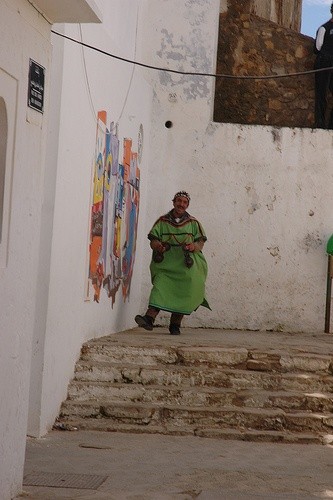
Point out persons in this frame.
[134,190,212,335]
[310,2,333,130]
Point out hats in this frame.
[173,190,190,202]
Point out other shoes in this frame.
[135,314,154,331]
[168,321,181,335]
